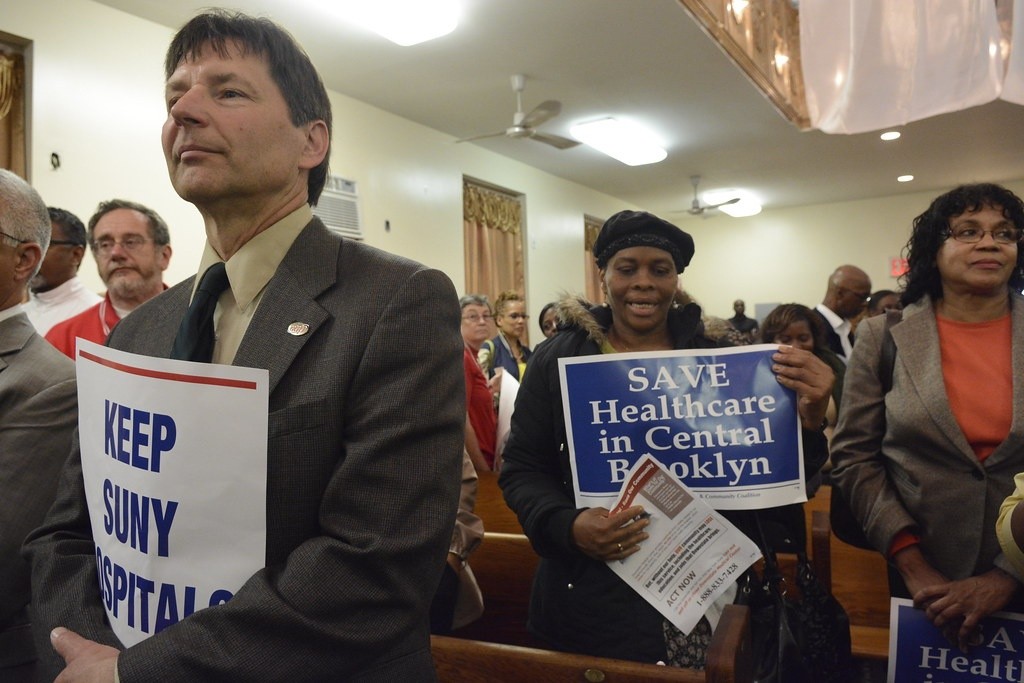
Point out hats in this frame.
[592,210,695,274]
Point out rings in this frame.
[617,543,623,553]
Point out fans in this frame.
[453,73,582,151]
[666,172,741,217]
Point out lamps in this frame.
[571,114,669,168]
[702,187,762,218]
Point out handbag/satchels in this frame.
[733,506,853,683]
[830,308,903,553]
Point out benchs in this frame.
[432,463,892,683]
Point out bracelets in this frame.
[818,417,829,433]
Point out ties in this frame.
[168,261,230,363]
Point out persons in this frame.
[0,168,77,683]
[45,199,172,358]
[539,303,563,338]
[869,290,902,318]
[50,6,464,683]
[476,291,533,416]
[764,303,846,409]
[431,443,485,641]
[813,264,872,360]
[502,209,834,668]
[23,206,105,337]
[730,301,757,336]
[825,182,1024,642]
[458,293,497,474]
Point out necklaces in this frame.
[619,342,631,352]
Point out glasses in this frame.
[507,313,529,320]
[462,313,495,322]
[93,236,155,250]
[948,225,1024,244]
